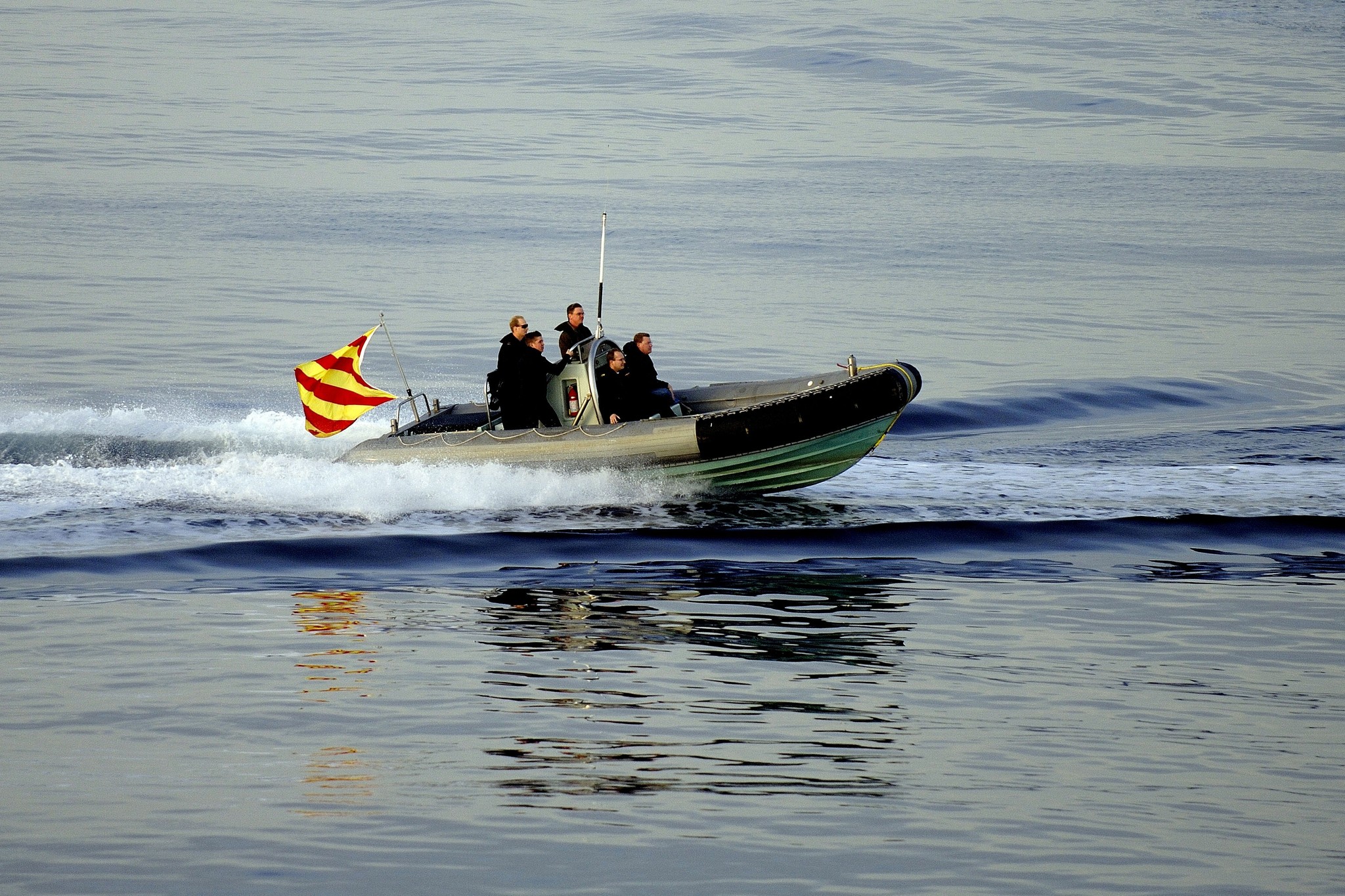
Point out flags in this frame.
[293,318,405,440]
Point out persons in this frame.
[559,303,593,364]
[597,332,694,425]
[496,315,573,431]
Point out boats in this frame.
[330,360,924,502]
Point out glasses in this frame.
[572,312,584,316]
[615,358,626,361]
[641,342,652,345]
[520,324,528,328]
